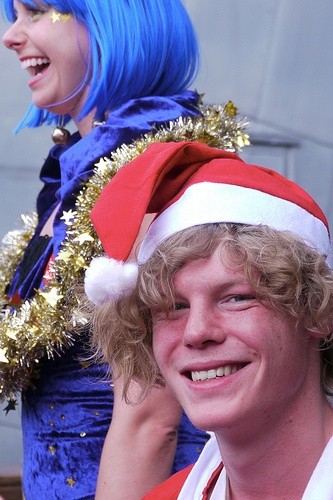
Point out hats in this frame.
[84,142,331,305]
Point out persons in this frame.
[83,141,332,500]
[0,0,252,500]
